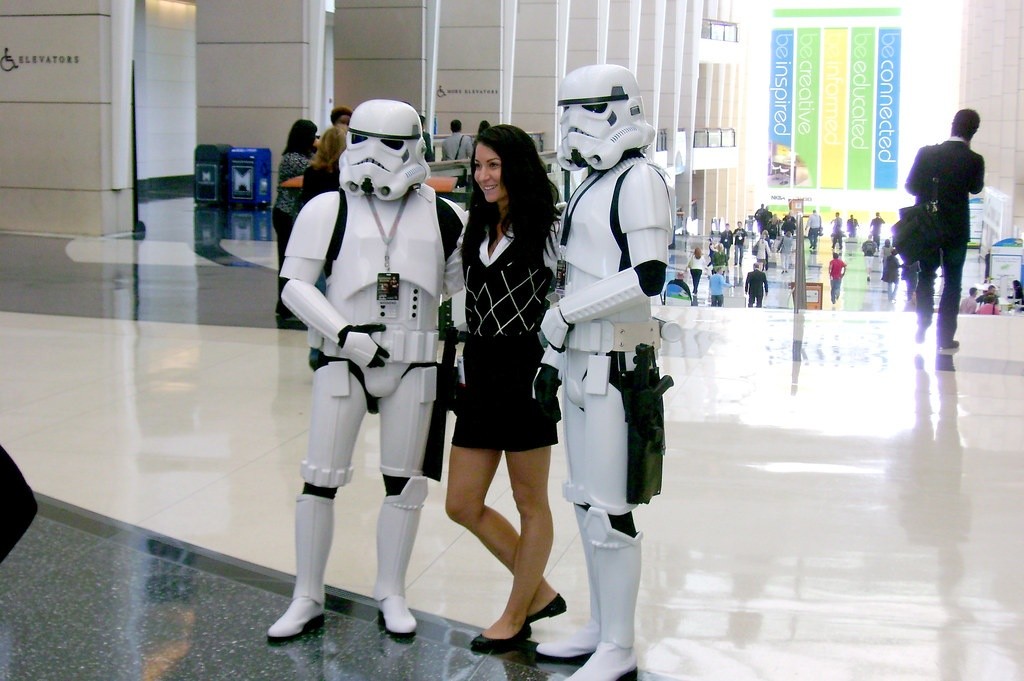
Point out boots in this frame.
[564,506,643,681]
[269,494,336,640]
[375,476,430,634]
[536,503,605,658]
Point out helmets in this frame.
[555,64,656,172]
[339,99,430,201]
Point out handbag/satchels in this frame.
[818,226,823,236]
[776,244,784,252]
[892,199,942,266]
[752,241,760,255]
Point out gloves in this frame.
[541,305,574,354]
[535,366,562,423]
[338,324,389,368]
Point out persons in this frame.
[478,120,491,134]
[829,252,847,304]
[719,223,733,255]
[560,267,565,285]
[862,234,878,284]
[847,215,858,237]
[870,212,885,251]
[530,63,674,681]
[831,212,842,249]
[882,239,1024,315]
[732,221,747,267]
[805,210,822,250]
[265,96,468,639]
[904,108,985,355]
[441,120,474,188]
[664,271,692,301]
[419,112,434,161]
[688,248,709,297]
[707,238,730,276]
[443,123,566,650]
[745,263,768,308]
[272,105,355,316]
[390,276,399,300]
[753,203,797,274]
[709,266,735,306]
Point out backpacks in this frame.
[865,242,874,256]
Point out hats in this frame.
[717,243,724,252]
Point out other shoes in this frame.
[275,301,295,319]
[916,316,933,343]
[471,622,532,648]
[938,339,960,349]
[525,592,567,623]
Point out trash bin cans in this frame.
[192,144,270,208]
